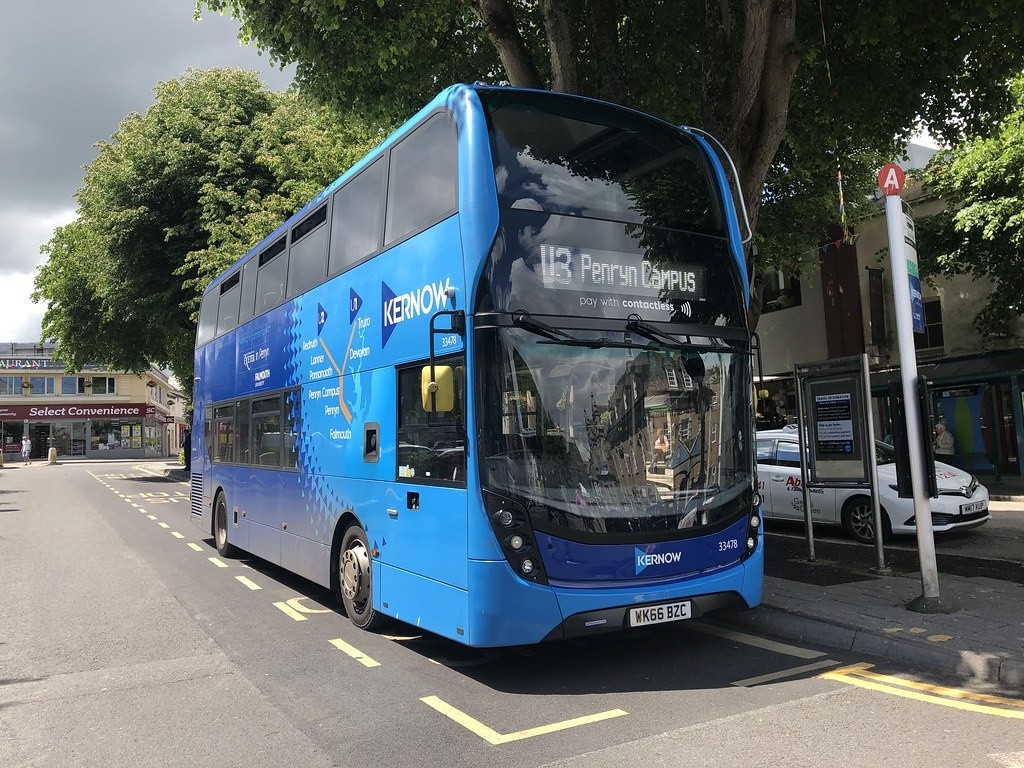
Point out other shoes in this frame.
[184,467,190,471]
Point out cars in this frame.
[755,425,993,543]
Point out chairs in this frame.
[397,427,574,493]
[261,432,299,469]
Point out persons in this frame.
[181,429,191,471]
[933,424,955,465]
[649,429,669,473]
[21,436,32,465]
[787,416,794,425]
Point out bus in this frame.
[189,81,766,648]
